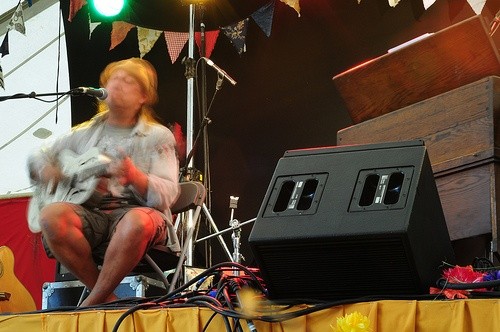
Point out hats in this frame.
[100,59,156,96]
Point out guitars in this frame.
[26,149,131,234]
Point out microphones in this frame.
[78,87,108,101]
[204,57,238,86]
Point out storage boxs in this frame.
[332,15,500,241]
[41,275,171,312]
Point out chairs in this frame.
[76,180,233,307]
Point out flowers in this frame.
[331,313,377,332]
[440,265,500,299]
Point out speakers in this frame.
[246,139,456,307]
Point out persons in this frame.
[26,58,182,308]
